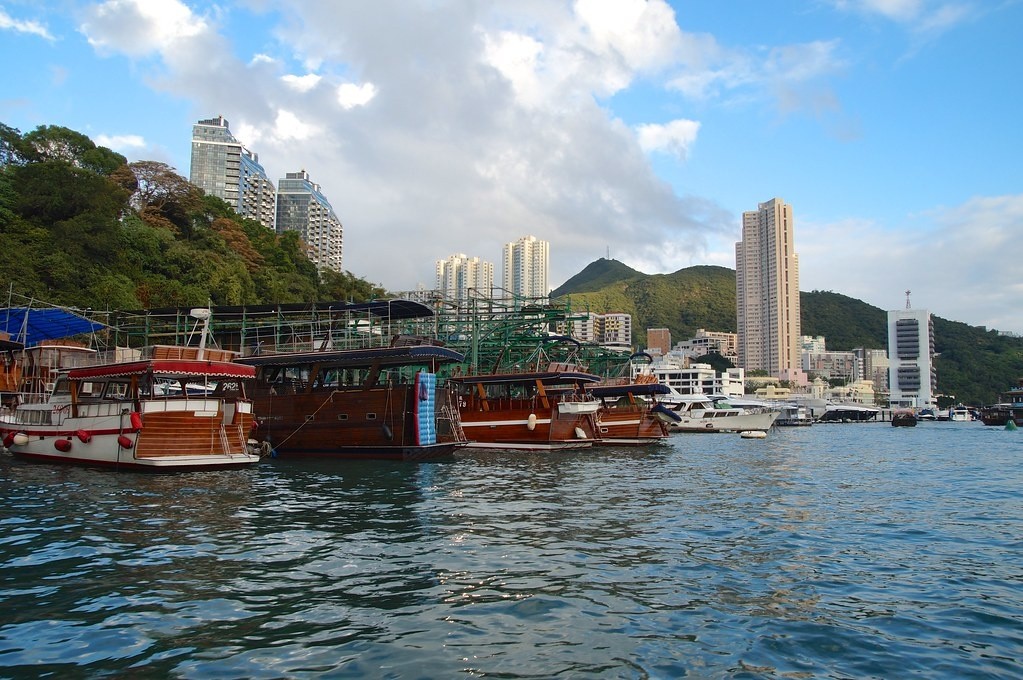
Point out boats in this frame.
[892,409,917,428]
[0,298,262,473]
[116,297,880,461]
[918,387,1023,426]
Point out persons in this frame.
[391,368,408,384]
[441,364,473,389]
[894,414,910,418]
